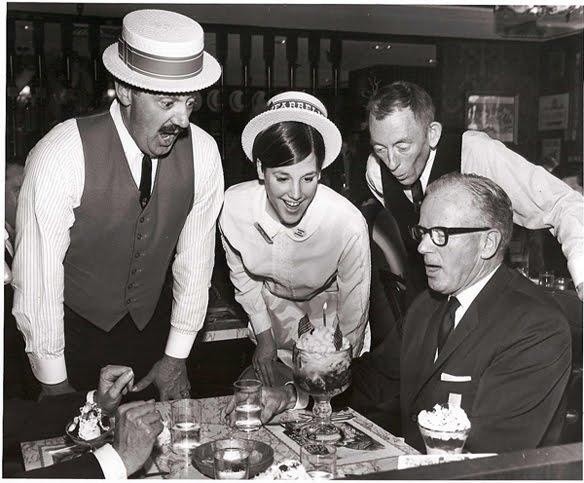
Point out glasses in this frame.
[407,224,492,247]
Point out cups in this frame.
[170,397,200,457]
[539,272,555,289]
[299,444,339,479]
[416,423,471,456]
[233,379,263,429]
[214,448,247,479]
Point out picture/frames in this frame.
[536,90,571,133]
[539,136,563,161]
[464,90,519,146]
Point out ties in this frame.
[410,178,424,207]
[437,295,461,355]
[139,154,152,210]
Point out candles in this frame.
[322,302,327,327]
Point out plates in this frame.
[191,438,275,478]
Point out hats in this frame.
[102,9,222,94]
[241,90,343,171]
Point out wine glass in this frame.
[293,343,352,445]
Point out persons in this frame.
[329,172,574,458]
[2,13,226,481]
[217,91,372,411]
[364,79,583,309]
